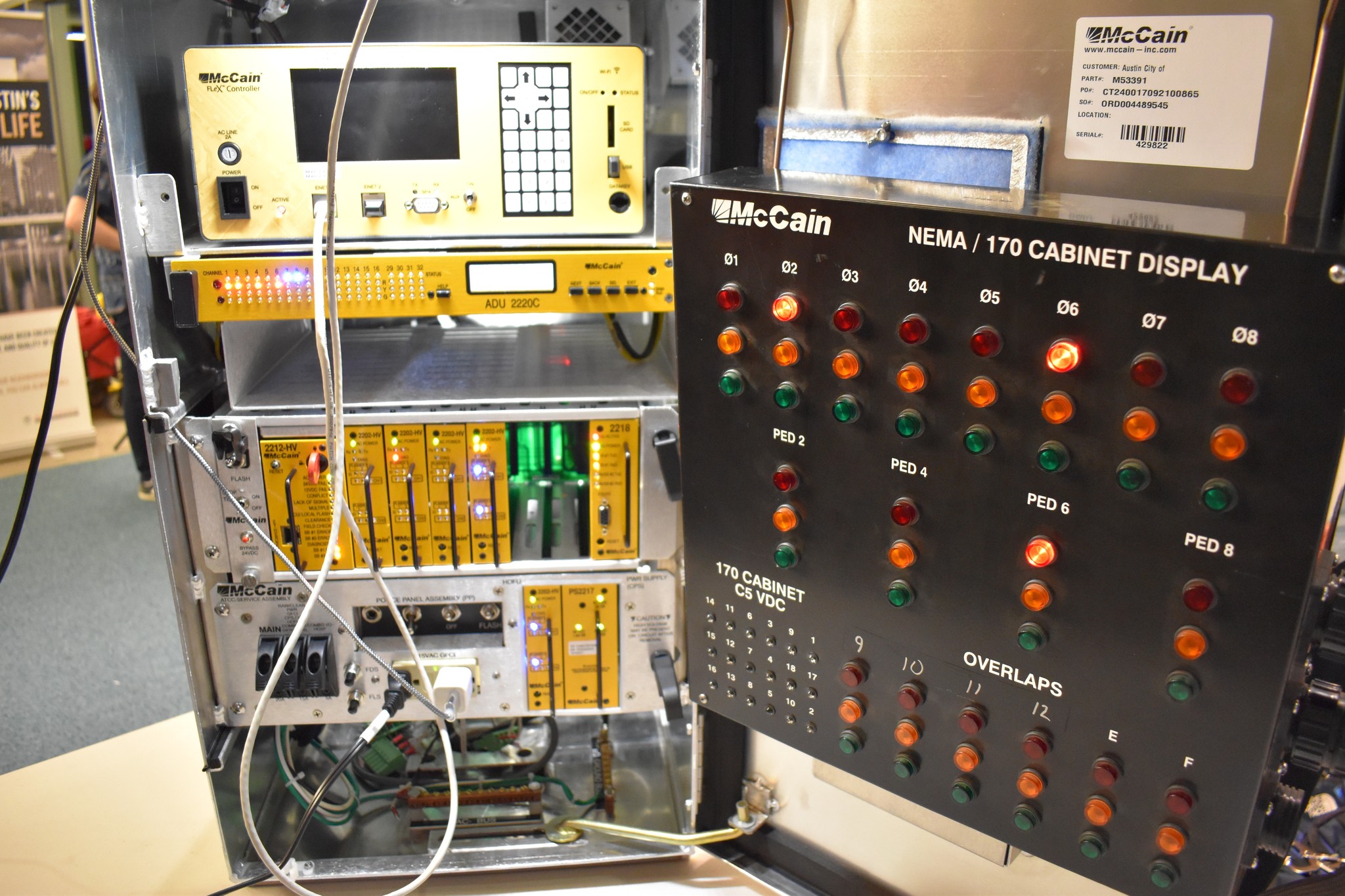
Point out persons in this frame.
[63,75,159,502]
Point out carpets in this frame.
[0,454,194,777]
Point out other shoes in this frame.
[138,483,156,502]
